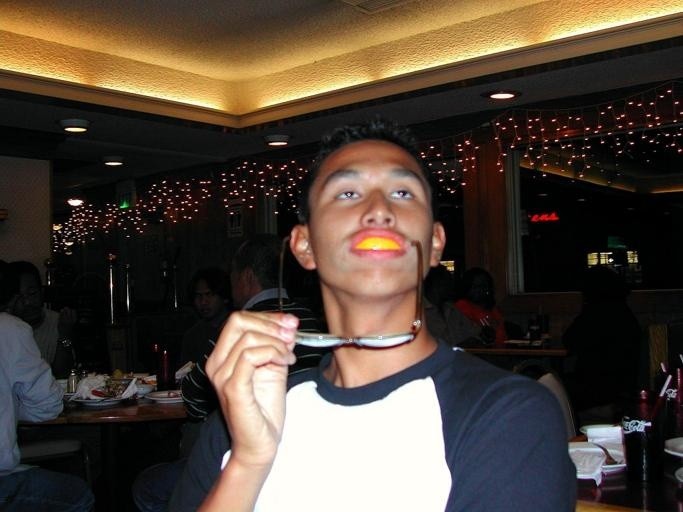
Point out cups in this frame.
[623,389,670,483]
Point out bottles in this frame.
[67,369,89,393]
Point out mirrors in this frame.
[504,123,683,293]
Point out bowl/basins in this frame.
[579,424,621,441]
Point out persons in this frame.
[423,263,512,373]
[563,268,638,412]
[1,237,326,512]
[167,129,578,512]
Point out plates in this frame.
[565,442,626,478]
[133,383,153,399]
[72,397,121,407]
[143,389,184,405]
[663,434,683,460]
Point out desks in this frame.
[13,329,683,512]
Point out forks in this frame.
[589,441,624,466]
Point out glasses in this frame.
[278,235,424,349]
